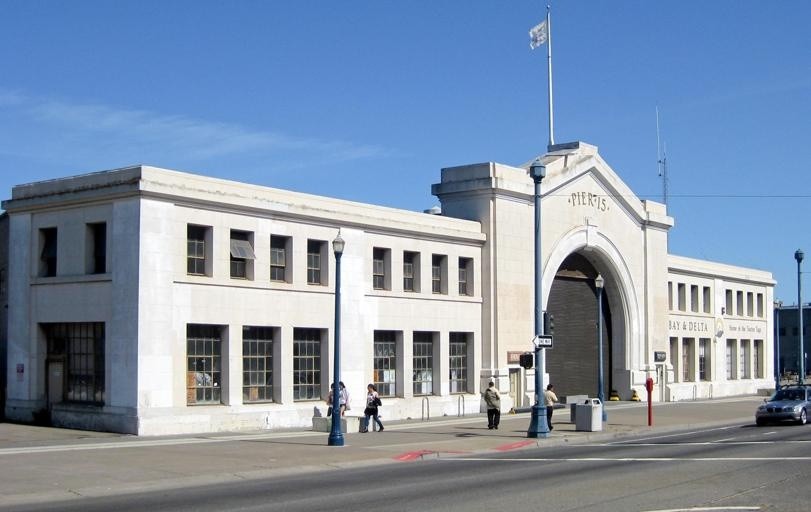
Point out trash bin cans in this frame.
[575,398,602,431]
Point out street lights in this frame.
[330,227,345,447]
[528,156,549,436]
[794,248,805,384]
[594,273,607,423]
[772,299,784,391]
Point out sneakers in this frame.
[362,425,384,432]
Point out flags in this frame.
[528,19,548,50]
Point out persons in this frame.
[483,381,501,429]
[339,381,352,417]
[362,383,384,433]
[544,384,559,431]
[326,383,333,416]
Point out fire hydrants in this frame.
[646,377,653,427]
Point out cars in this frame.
[757,384,811,425]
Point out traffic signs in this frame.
[533,336,552,350]
